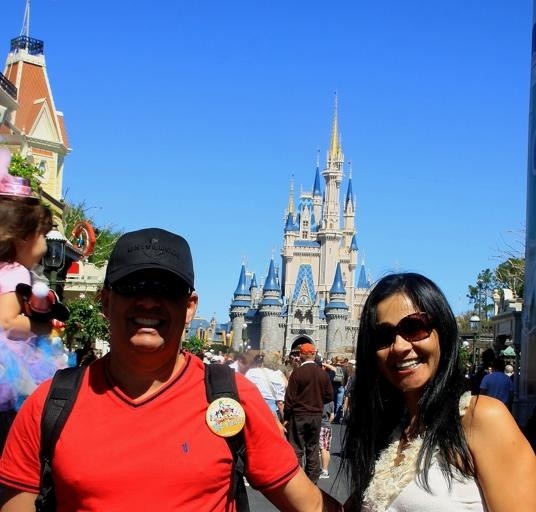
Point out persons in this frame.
[284,343,356,484]
[339,273,536,512]
[0,194,68,412]
[0,228,344,512]
[466,360,520,405]
[195,348,298,419]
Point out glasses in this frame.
[110,270,191,301]
[366,313,432,350]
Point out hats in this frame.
[300,342,313,356]
[106,229,194,293]
